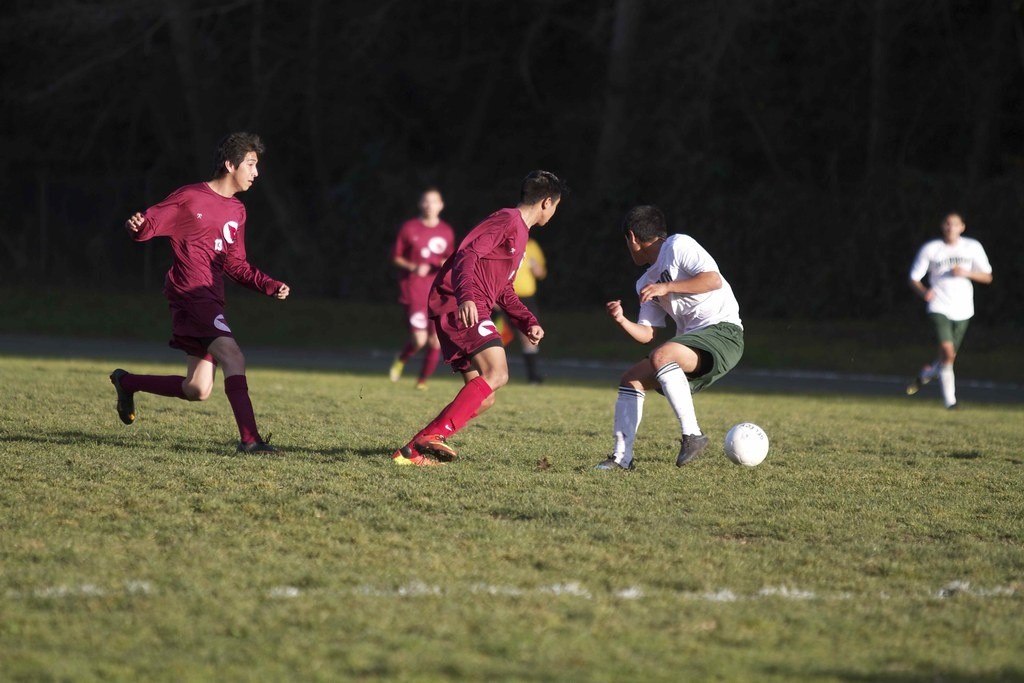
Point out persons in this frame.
[387,186,454,390]
[110,132,285,457]
[595,205,744,471]
[489,234,547,385]
[905,210,994,412]
[392,169,562,467]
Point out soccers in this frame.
[724,422,770,467]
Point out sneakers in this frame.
[236,432,279,455]
[414,434,457,462]
[906,366,933,396]
[947,403,963,410]
[390,449,447,467]
[676,429,710,466]
[389,352,405,382]
[110,368,136,425]
[415,383,427,390]
[594,454,636,471]
